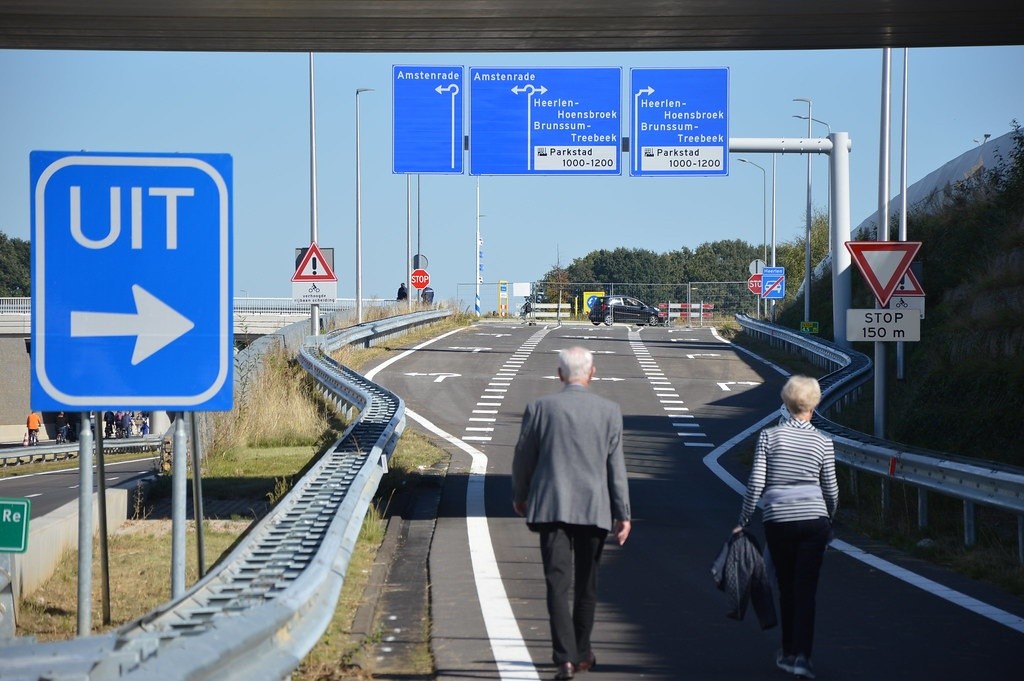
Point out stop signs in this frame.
[747,273,763,295]
[410,269,431,290]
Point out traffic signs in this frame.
[389,63,467,174]
[466,66,625,177]
[628,64,730,178]
[28,149,236,414]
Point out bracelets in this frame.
[738,521,752,528]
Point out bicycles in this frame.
[102,418,134,439]
[54,425,71,445]
[516,307,536,326]
[26,424,41,447]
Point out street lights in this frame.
[792,97,813,338]
[793,113,832,255]
[355,87,377,324]
[736,157,768,318]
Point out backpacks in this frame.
[525,304,531,312]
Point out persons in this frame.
[396,282,407,300]
[26,410,151,445]
[492,311,497,316]
[730,373,840,675]
[511,347,632,681]
[522,298,531,323]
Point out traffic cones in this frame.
[22,432,29,447]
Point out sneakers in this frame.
[794,658,816,680]
[776,656,794,672]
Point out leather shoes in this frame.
[575,651,595,672]
[554,663,574,680]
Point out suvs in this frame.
[588,294,663,327]
